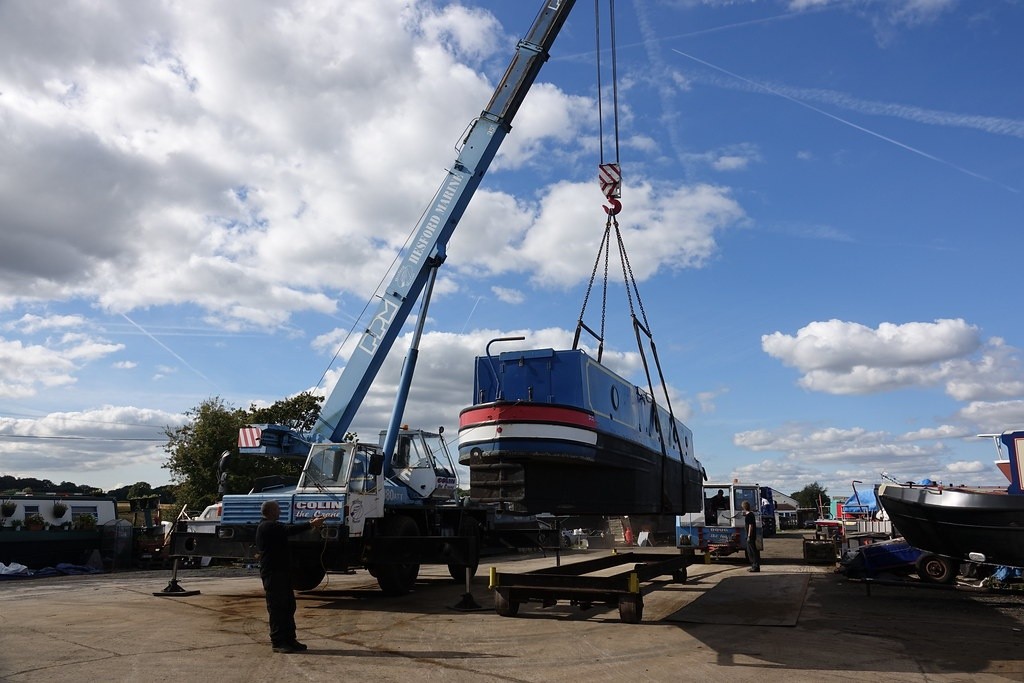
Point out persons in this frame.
[741,500,760,573]
[256,501,323,654]
[708,489,728,512]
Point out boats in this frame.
[873,483,1024,570]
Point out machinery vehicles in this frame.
[154,0,625,600]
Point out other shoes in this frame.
[747,568,760,572]
[290,639,307,652]
[271,643,295,653]
